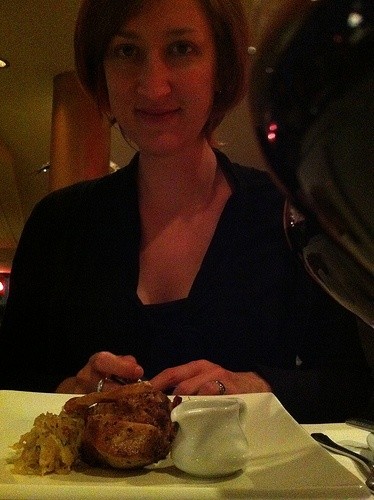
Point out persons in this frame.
[2,1,370,424]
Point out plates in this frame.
[1,389,373,499]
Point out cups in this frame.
[170,397,254,481]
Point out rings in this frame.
[213,379,226,395]
[96,377,109,392]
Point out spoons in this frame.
[310,431,374,495]
